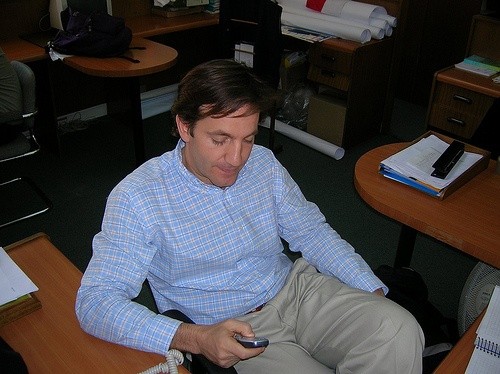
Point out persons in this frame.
[74,60,424,374]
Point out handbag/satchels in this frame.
[373,265,427,326]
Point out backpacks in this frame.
[51,10,132,57]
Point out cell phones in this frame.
[232,334,270,349]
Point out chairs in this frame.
[0,61,54,228]
[220,0,287,153]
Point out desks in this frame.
[0,13,219,165]
[352,142,500,374]
[0,232,191,374]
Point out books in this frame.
[0,247,41,324]
[453,54,500,77]
[465,285,500,374]
[375,136,485,200]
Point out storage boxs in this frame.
[306,96,356,146]
[140,76,182,119]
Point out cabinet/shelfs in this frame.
[308,37,393,130]
[424,65,500,142]
